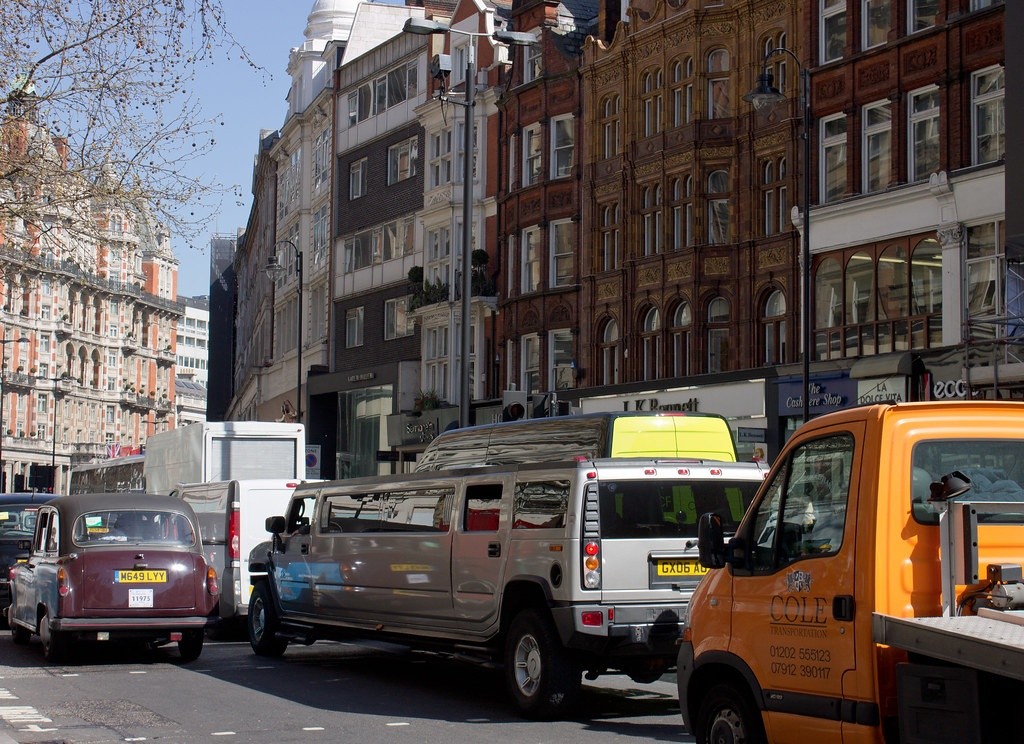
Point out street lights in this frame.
[403,17,538,429]
[50,377,76,493]
[260,240,304,423]
[0,331,30,493]
[742,48,813,497]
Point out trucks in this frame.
[142,422,305,495]
[677,401,1024,744]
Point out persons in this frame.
[291,525,310,536]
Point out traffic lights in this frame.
[48,484,53,494]
[502,383,528,422]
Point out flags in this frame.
[107,444,120,458]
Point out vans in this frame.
[414,411,739,475]
[247,458,771,711]
[168,479,332,640]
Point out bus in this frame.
[68,455,148,524]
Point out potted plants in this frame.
[413,384,441,411]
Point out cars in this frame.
[0,493,220,663]
